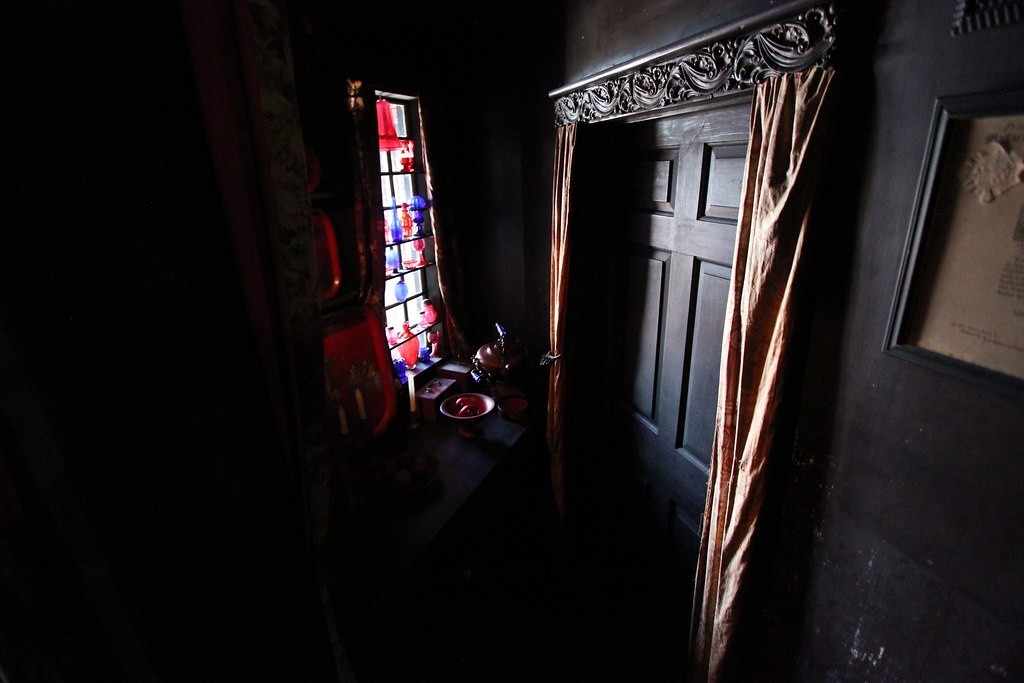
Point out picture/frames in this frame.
[882,82,1023,402]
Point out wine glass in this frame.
[426,331,441,358]
[415,239,427,265]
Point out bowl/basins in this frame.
[401,260,418,269]
[498,395,528,421]
[385,267,394,278]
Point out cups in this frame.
[402,139,415,172]
[394,359,408,379]
[420,347,430,362]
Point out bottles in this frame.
[396,323,419,370]
[389,195,412,242]
[386,327,398,345]
[418,299,437,328]
[387,246,400,269]
[396,280,408,303]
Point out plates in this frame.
[443,394,495,437]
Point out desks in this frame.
[320,417,530,605]
